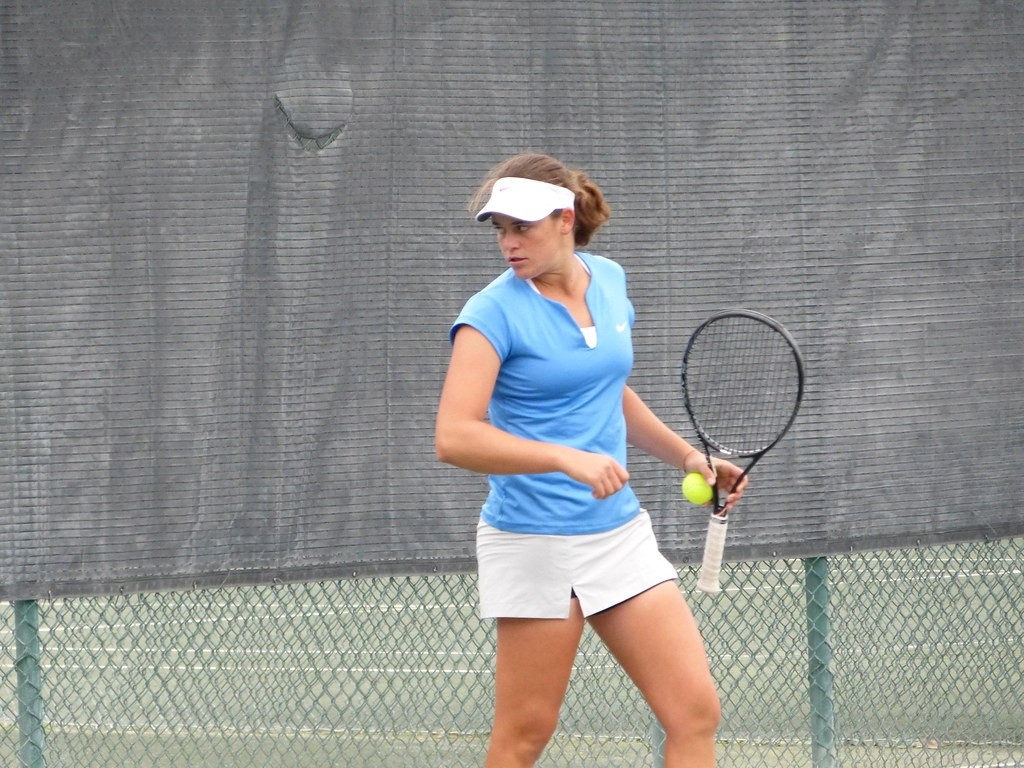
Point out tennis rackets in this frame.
[679,310,806,594]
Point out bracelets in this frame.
[679,449,701,473]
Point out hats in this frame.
[474,177,575,223]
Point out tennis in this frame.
[683,473,712,506]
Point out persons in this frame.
[435,154,751,768]
